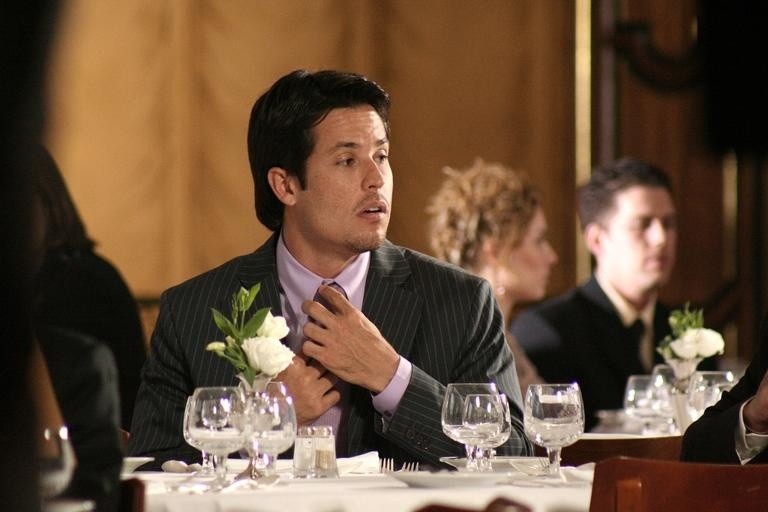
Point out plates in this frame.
[390,465,527,488]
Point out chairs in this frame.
[556,436,692,472]
[586,457,767,510]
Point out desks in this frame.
[119,458,594,511]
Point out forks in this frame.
[380,456,420,475]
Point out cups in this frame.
[623,363,734,435]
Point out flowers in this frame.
[205,282,296,393]
[656,300,725,381]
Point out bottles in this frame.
[293,425,339,478]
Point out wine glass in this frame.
[462,392,513,473]
[182,380,298,482]
[524,381,586,478]
[441,380,498,472]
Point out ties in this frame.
[629,320,644,351]
[309,281,348,314]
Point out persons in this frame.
[423,158,556,424]
[0,139,147,437]
[511,157,721,434]
[128,66,533,471]
[679,336,767,467]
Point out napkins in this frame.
[226,451,380,478]
